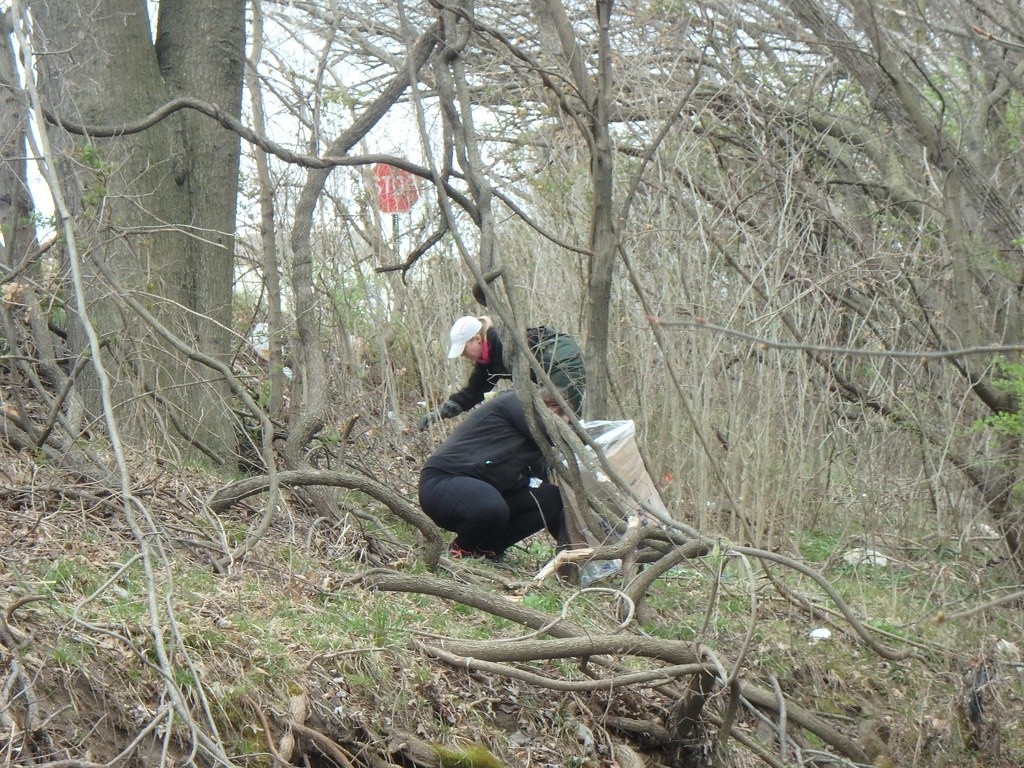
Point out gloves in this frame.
[420,400,462,432]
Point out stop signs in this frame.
[372,160,424,215]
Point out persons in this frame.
[418,386,572,558]
[417,316,587,433]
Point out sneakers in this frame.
[448,540,514,575]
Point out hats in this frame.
[447,316,482,359]
